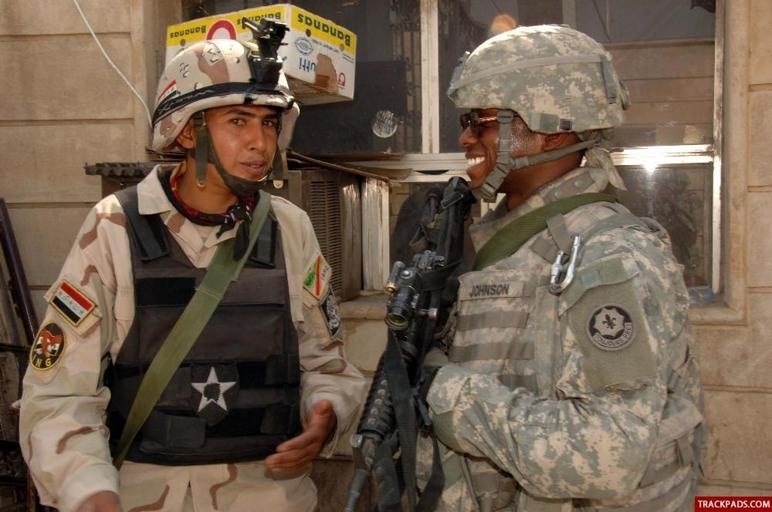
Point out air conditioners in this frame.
[101,169,362,304]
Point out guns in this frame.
[344,176,477,512]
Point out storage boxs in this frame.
[165,3,357,106]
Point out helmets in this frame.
[149,38,300,154]
[447,23,632,136]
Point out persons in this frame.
[17,35,368,511]
[412,21,707,511]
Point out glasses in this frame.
[459,112,521,138]
